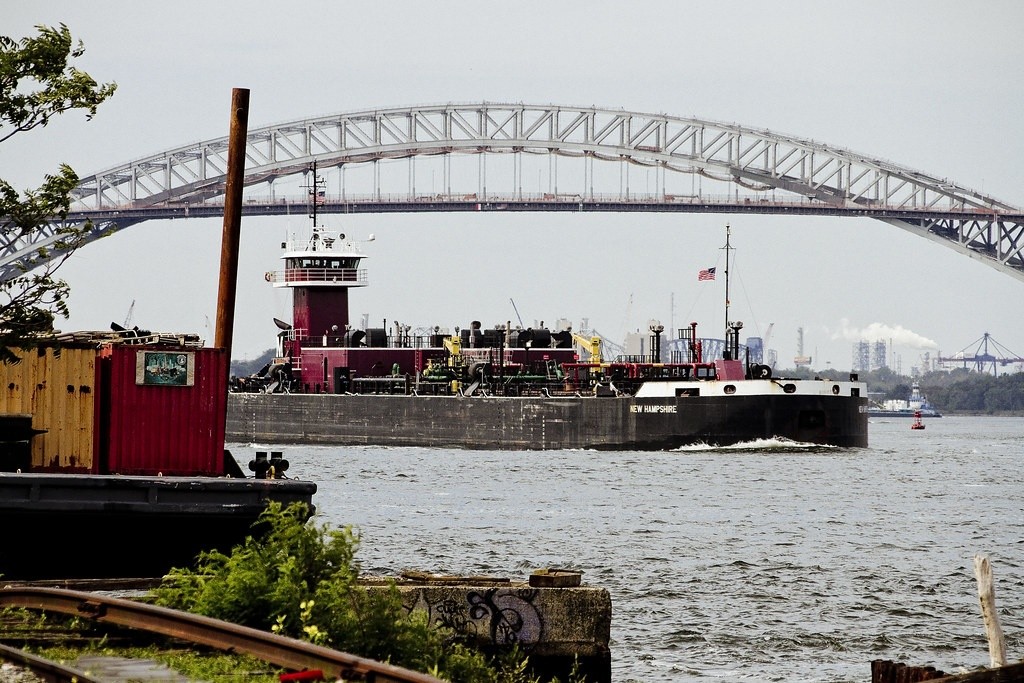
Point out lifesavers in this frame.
[265,272,270,281]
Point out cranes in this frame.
[763,323,775,352]
[123,300,135,331]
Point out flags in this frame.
[698,267,716,281]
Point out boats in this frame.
[0,86,316,580]
[867,376,942,417]
[225,161,869,452]
[912,408,926,429]
